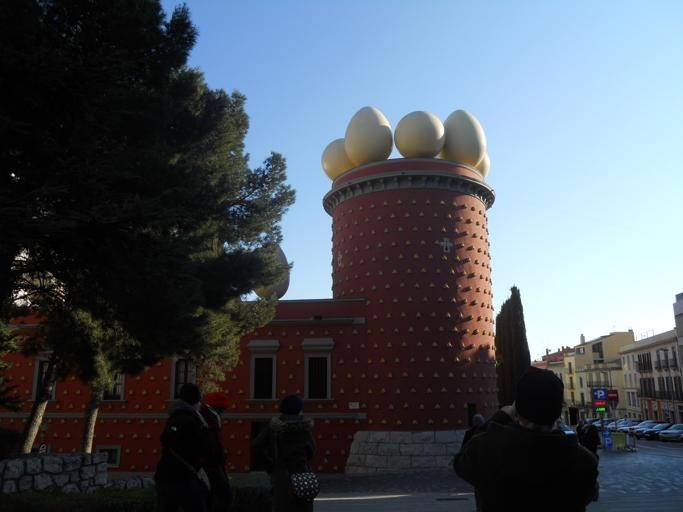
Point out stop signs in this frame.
[607,389,618,401]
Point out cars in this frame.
[561,423,578,443]
[582,416,682,444]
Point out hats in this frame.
[200,391,232,411]
[512,364,565,427]
[276,394,304,414]
[173,382,201,405]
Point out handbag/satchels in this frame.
[284,469,322,504]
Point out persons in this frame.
[451,368,599,512]
[270,394,319,512]
[581,419,601,461]
[151,382,213,511]
[576,419,583,445]
[460,412,485,449]
[196,392,237,512]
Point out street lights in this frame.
[632,359,646,419]
[599,365,620,451]
[657,347,677,423]
